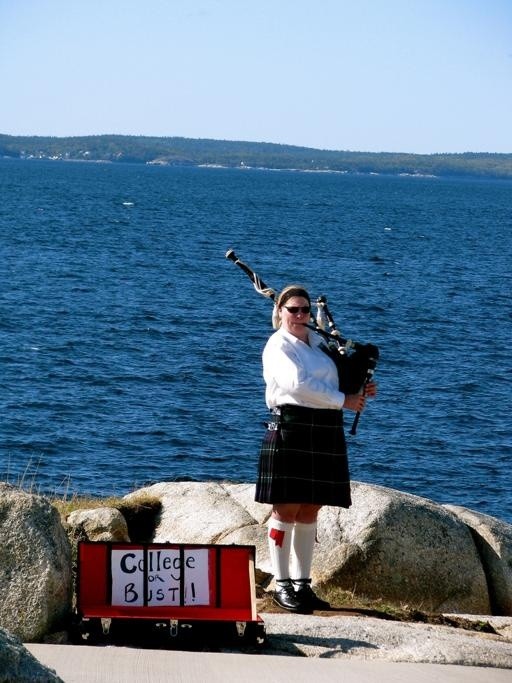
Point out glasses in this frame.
[282,304,311,314]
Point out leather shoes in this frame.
[272,583,330,614]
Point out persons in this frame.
[255,287,376,611]
